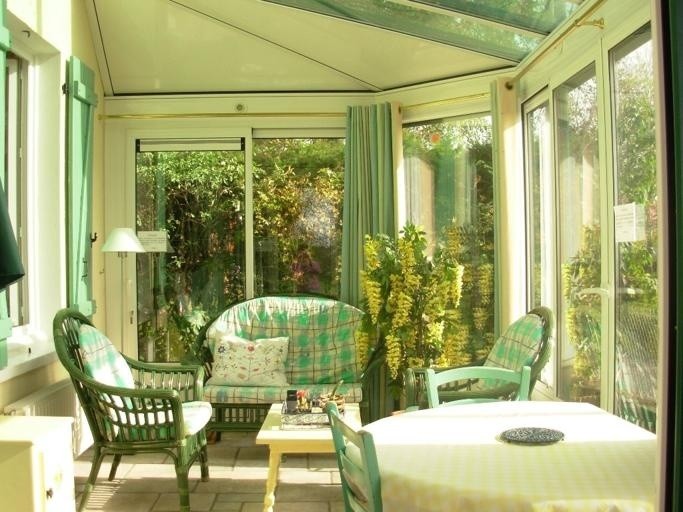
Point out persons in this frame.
[292,251,322,293]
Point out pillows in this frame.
[212,328,291,386]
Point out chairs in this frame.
[403,306,552,411]
[322,400,382,511]
[52,306,213,510]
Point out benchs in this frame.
[196,295,381,443]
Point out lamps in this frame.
[99,227,147,356]
